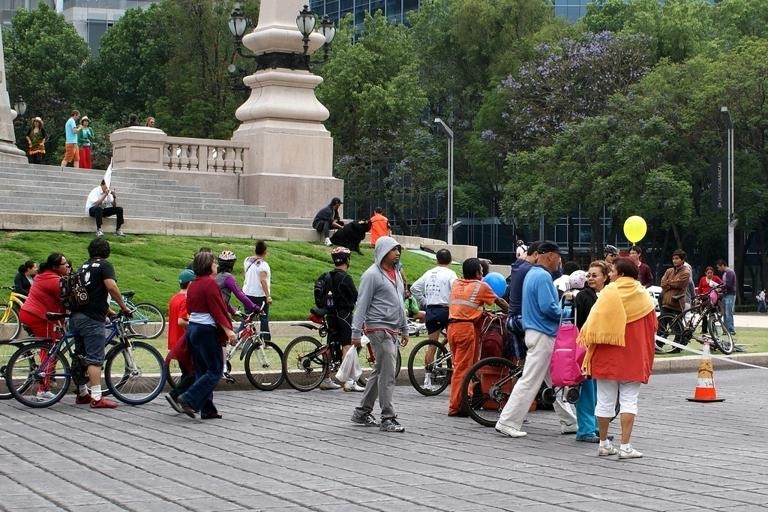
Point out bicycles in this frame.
[0,284,38,345]
[171,309,284,391]
[652,293,734,354]
[279,310,400,392]
[463,330,525,427]
[106,289,164,339]
[407,314,504,395]
[6,310,166,407]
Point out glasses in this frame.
[586,274,599,278]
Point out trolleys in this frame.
[539,292,586,404]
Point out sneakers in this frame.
[75,394,91,404]
[576,435,599,443]
[344,382,366,392]
[165,392,183,412]
[598,445,618,456]
[494,420,527,437]
[324,239,332,246]
[90,396,118,408]
[561,423,577,433]
[35,391,56,402]
[177,399,196,418]
[116,230,126,237]
[380,417,405,432]
[351,410,380,427]
[618,448,642,458]
[96,229,104,237]
[320,382,341,389]
[201,413,222,419]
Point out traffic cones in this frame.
[686,338,725,404]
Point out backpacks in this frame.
[481,316,504,367]
[60,258,89,309]
[314,271,335,309]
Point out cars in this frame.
[742,283,753,300]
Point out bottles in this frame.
[327,291,334,307]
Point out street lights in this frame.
[716,105,739,315]
[431,118,456,244]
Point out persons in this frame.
[329,219,372,254]
[397,234,738,458]
[78,114,96,168]
[124,113,141,128]
[86,176,125,239]
[24,116,50,162]
[13,236,134,410]
[365,206,392,248]
[348,235,410,434]
[163,240,276,419]
[316,245,367,395]
[146,115,155,128]
[751,280,768,313]
[312,196,348,245]
[60,106,81,167]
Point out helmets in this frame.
[218,250,237,260]
[331,247,352,259]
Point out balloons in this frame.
[621,214,648,251]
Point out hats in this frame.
[79,116,91,123]
[605,245,620,255]
[538,241,569,255]
[32,117,44,127]
[178,269,197,283]
[569,270,587,289]
